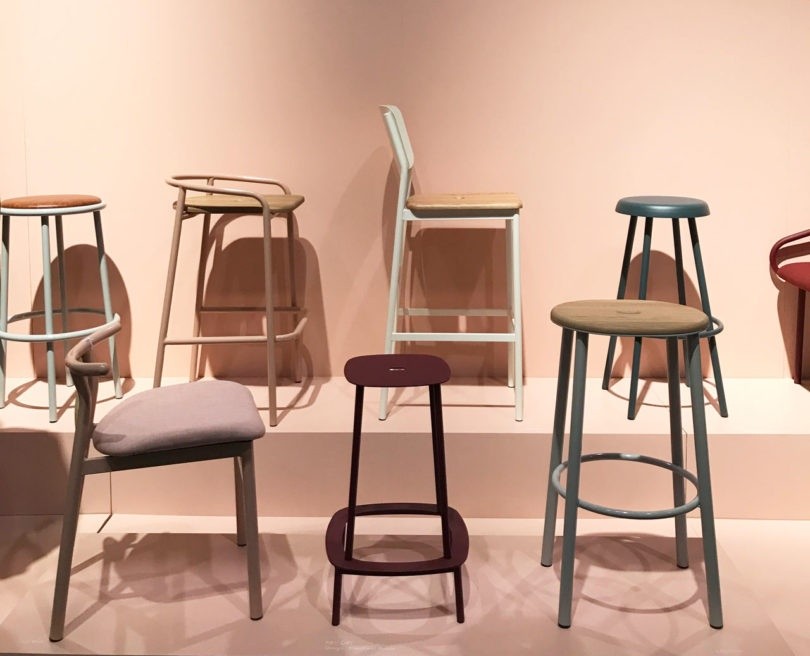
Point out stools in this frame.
[48,320,267,641]
[0,194,122,423]
[151,172,308,426]
[600,195,730,420]
[323,352,470,625]
[770,229,810,384]
[540,299,725,630]
[378,103,523,422]
